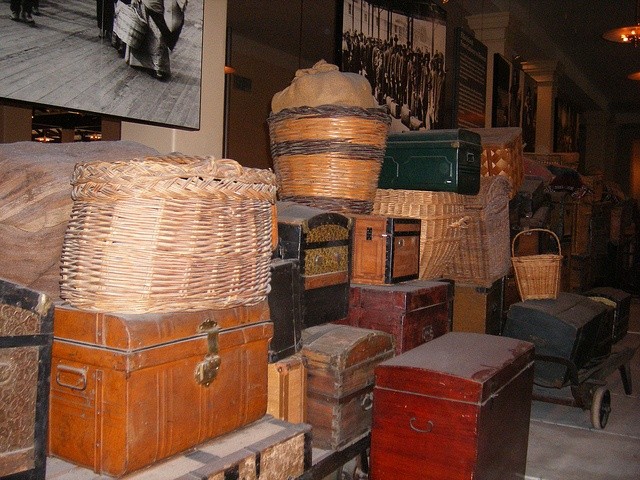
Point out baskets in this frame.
[512,228,564,302]
[583,174,601,200]
[60,158,278,309]
[112,1,148,51]
[372,190,472,282]
[267,109,391,214]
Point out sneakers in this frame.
[22,13,33,27]
[8,9,19,22]
[32,7,40,15]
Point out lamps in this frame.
[602,19,640,50]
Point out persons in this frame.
[21,0,40,19]
[557,116,576,152]
[124,0,187,82]
[341,30,446,131]
[9,0,35,22]
[523,87,535,151]
[97,0,131,58]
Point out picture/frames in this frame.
[0,0,204,132]
[336,0,447,129]
[491,54,539,154]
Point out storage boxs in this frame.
[0,280,53,479]
[368,332,536,480]
[275,200,353,328]
[268,259,304,363]
[343,213,421,285]
[300,324,396,453]
[48,301,276,477]
[204,356,312,479]
[325,280,451,357]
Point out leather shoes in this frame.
[152,69,168,81]
